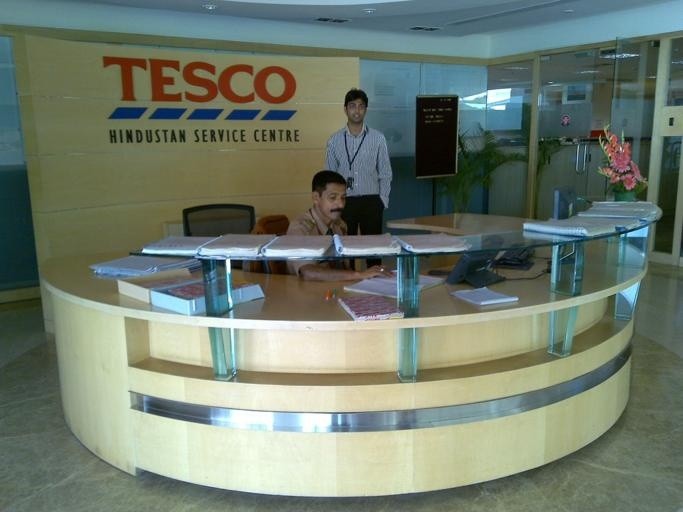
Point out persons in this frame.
[280,168,396,283]
[324,87,394,270]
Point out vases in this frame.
[615,188,636,203]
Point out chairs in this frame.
[181,202,290,276]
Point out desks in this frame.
[37,194,661,499]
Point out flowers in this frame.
[596,128,647,192]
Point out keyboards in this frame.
[497,241,536,265]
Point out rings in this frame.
[378,268,385,272]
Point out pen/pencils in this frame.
[325,287,337,301]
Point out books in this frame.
[339,294,406,323]
[85,254,204,281]
[341,268,447,298]
[447,284,520,308]
[520,199,657,238]
